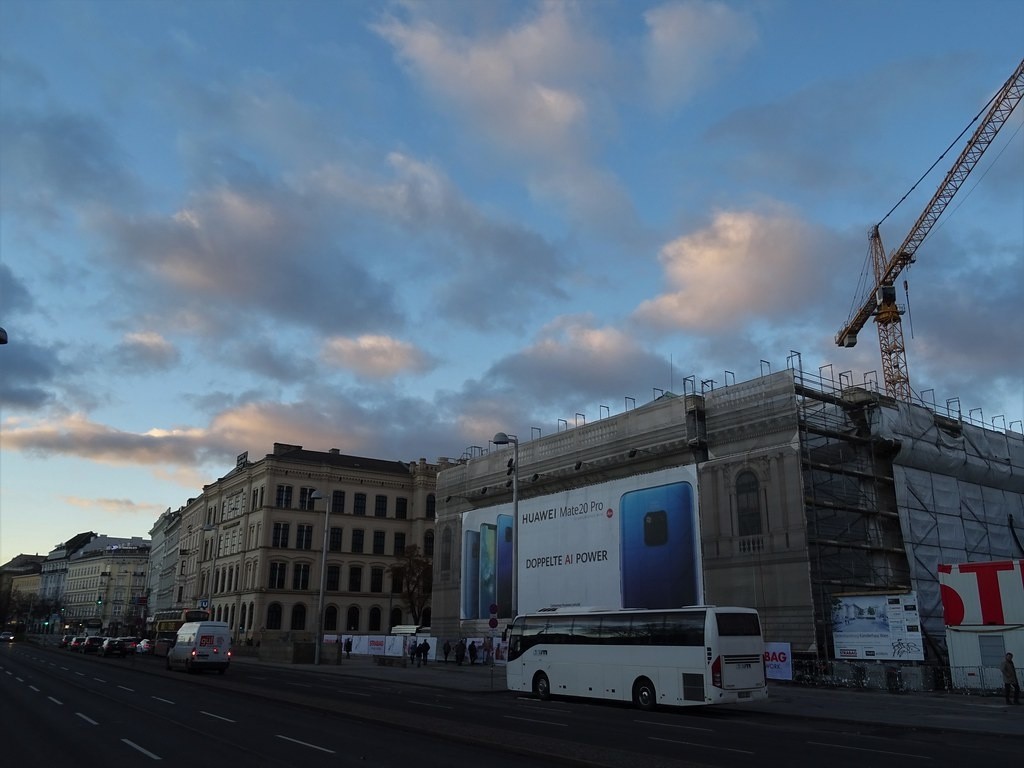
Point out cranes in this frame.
[834,58,1024,404]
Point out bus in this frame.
[82,618,109,637]
[502,606,769,710]
[151,609,211,656]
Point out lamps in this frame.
[445,496,466,502]
[629,448,659,458]
[532,474,549,481]
[575,461,601,470]
[506,480,524,487]
[481,487,502,494]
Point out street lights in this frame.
[311,490,330,641]
[203,525,220,610]
[494,432,520,621]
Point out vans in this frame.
[167,621,230,674]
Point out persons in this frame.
[496,643,507,660]
[443,640,451,664]
[346,638,352,659]
[85,633,88,637]
[106,633,109,637]
[410,639,430,668]
[455,640,466,666]
[1001,653,1022,705]
[468,641,477,664]
[95,632,99,636]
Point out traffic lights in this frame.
[97,600,102,605]
[60,608,65,613]
[44,620,49,626]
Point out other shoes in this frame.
[1006,700,1012,705]
[1014,699,1021,705]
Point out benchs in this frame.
[375,656,411,668]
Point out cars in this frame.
[68,637,86,652]
[0,632,14,641]
[136,638,154,654]
[61,635,76,648]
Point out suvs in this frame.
[78,636,104,655]
[96,639,127,659]
[117,636,137,655]
